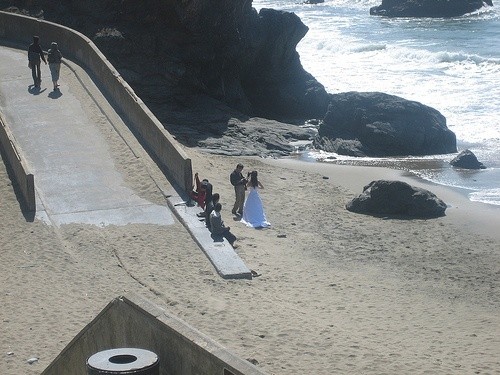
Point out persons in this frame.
[230,164,247,216]
[47,41,61,91]
[28,36,47,90]
[241,171,271,227]
[190,172,236,242]
